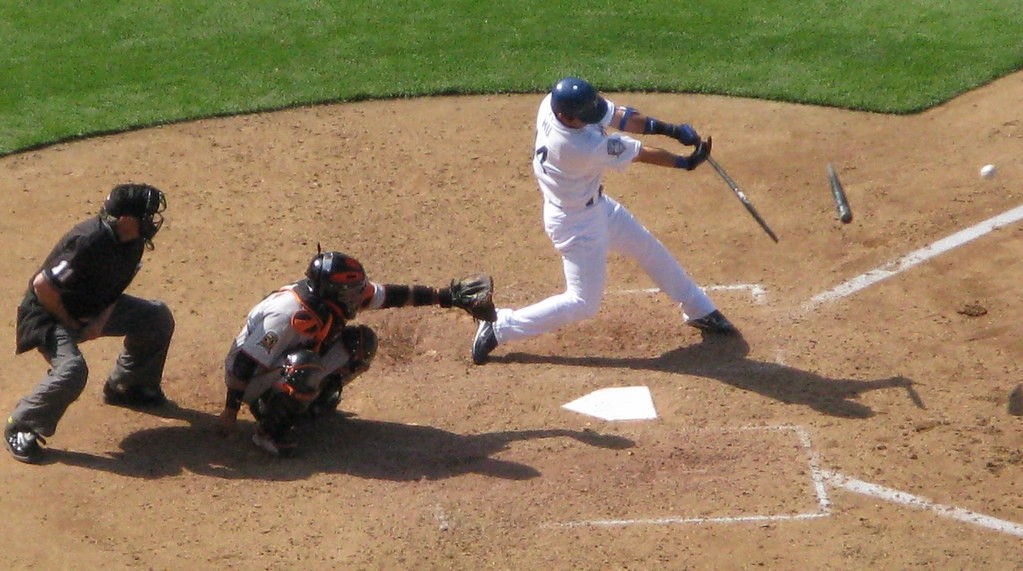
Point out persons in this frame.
[220,251,498,460]
[472,78,735,365]
[3,183,175,464]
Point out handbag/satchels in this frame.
[15,305,57,355]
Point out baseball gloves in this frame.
[439,273,498,323]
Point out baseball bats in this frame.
[695,143,780,244]
[826,161,853,224]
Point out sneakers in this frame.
[3,419,46,462]
[472,308,499,365]
[687,309,737,333]
[252,436,294,459]
[310,402,336,418]
[103,377,178,412]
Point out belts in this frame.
[549,185,601,211]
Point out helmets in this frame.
[304,251,367,320]
[550,77,608,124]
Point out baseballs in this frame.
[981,164,997,180]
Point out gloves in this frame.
[667,123,700,146]
[684,136,713,171]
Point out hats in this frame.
[104,184,159,217]
